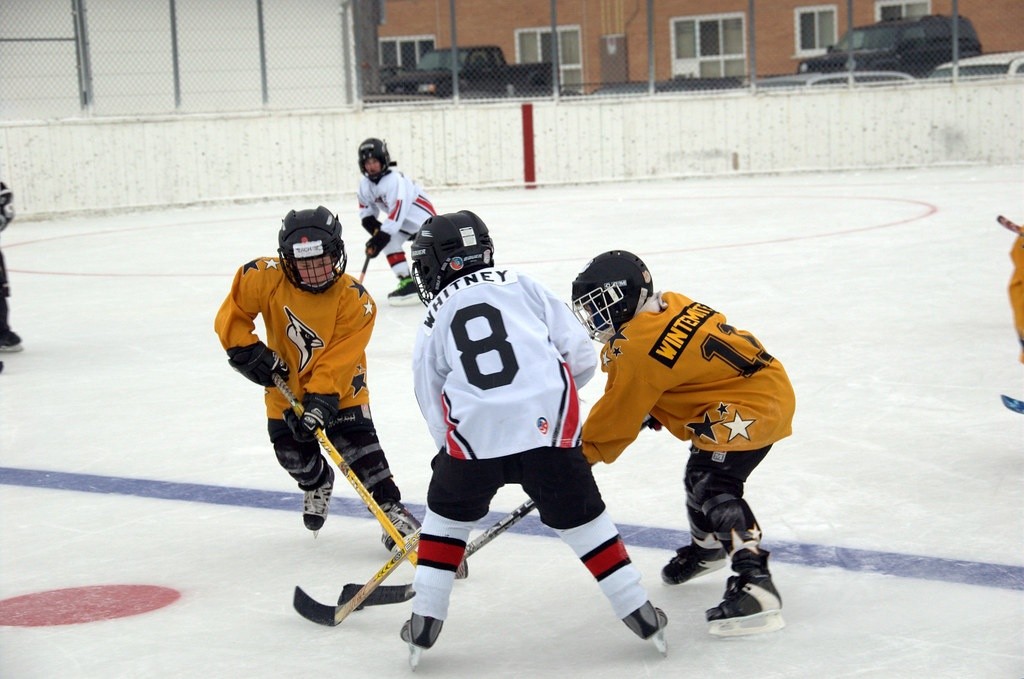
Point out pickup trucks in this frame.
[380,43,553,97]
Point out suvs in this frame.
[797,11,983,79]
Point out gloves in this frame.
[362,214,382,237]
[281,393,340,443]
[227,340,290,387]
[367,229,391,258]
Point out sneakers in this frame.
[402,610,443,671]
[622,603,669,653]
[704,550,785,637]
[388,272,422,305]
[366,500,421,556]
[298,458,334,541]
[0,328,21,353]
[660,540,728,594]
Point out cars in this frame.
[589,50,1024,95]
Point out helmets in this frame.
[279,207,345,293]
[571,250,653,346]
[357,140,389,178]
[410,210,495,305]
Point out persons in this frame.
[998,209,1024,369]
[0,181,23,375]
[357,138,438,306]
[214,206,423,552]
[400,209,668,669]
[564,247,795,638]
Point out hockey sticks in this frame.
[293,527,421,627]
[274,373,468,579]
[357,259,370,285]
[337,413,654,608]
